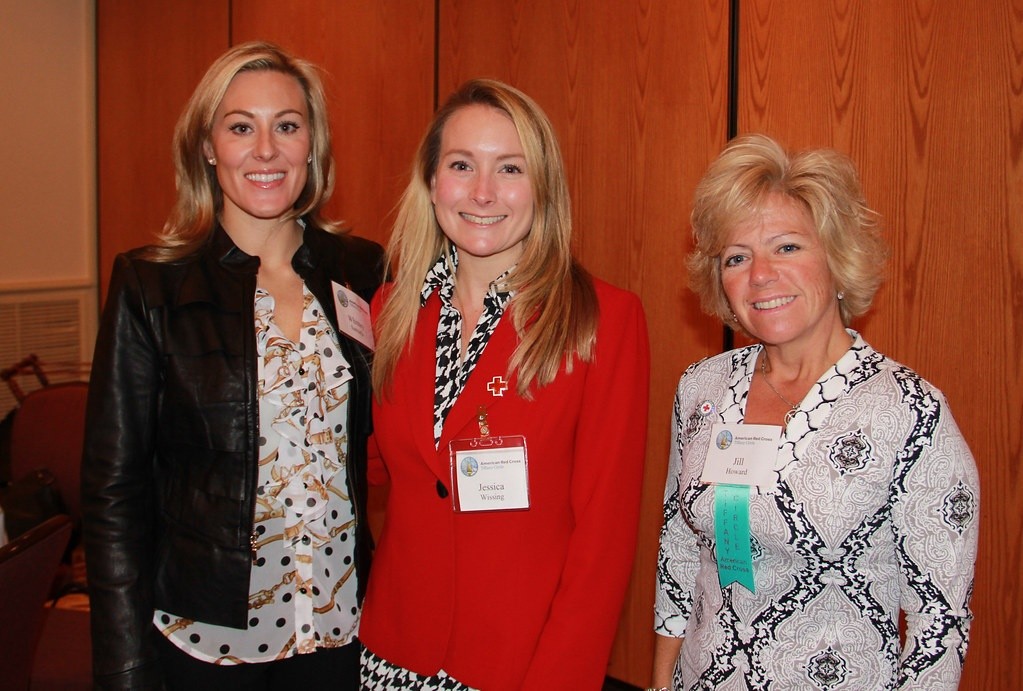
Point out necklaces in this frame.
[762,352,807,425]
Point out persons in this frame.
[645,133,980,691]
[80,39,393,691]
[356,78,651,691]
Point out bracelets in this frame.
[647,687,671,691]
